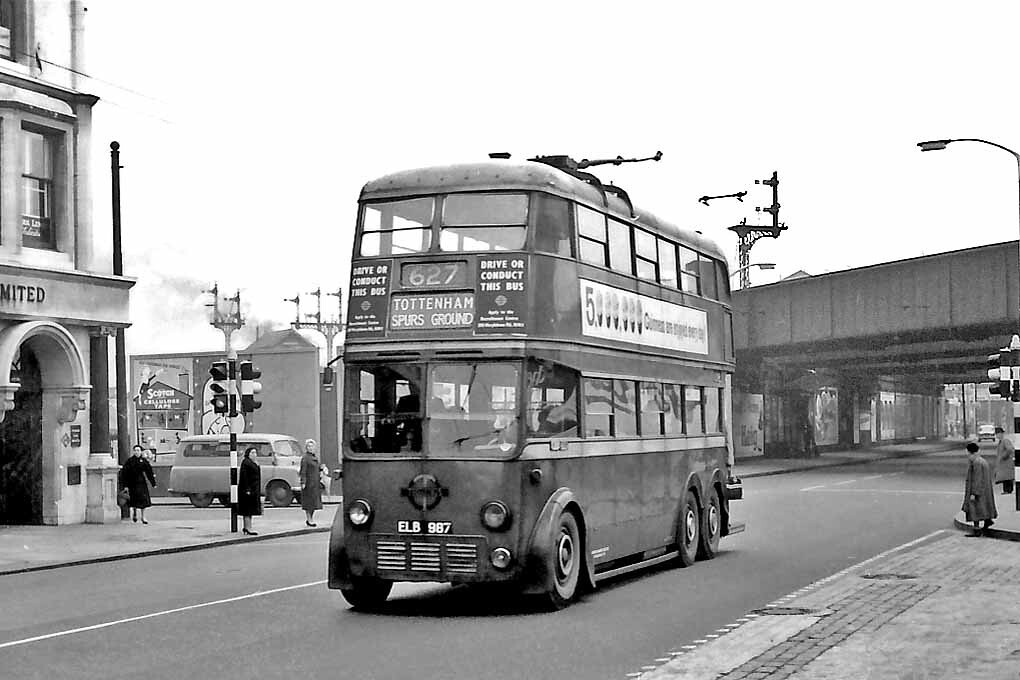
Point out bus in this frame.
[325,156,742,616]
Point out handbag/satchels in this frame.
[117,487,131,506]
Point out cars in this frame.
[978,424,996,442]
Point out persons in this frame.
[961,443,998,537]
[236,447,262,536]
[119,445,157,525]
[994,427,1016,495]
[298,439,325,527]
[394,378,444,452]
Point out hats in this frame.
[966,442,980,454]
[993,427,1009,434]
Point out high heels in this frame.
[243,528,248,535]
[246,530,259,535]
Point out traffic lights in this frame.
[987,352,1011,399]
[209,361,229,416]
[239,360,262,414]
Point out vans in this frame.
[165,431,305,509]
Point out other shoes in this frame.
[964,529,982,537]
[142,519,148,524]
[306,520,317,527]
[132,515,141,523]
[980,520,995,532]
